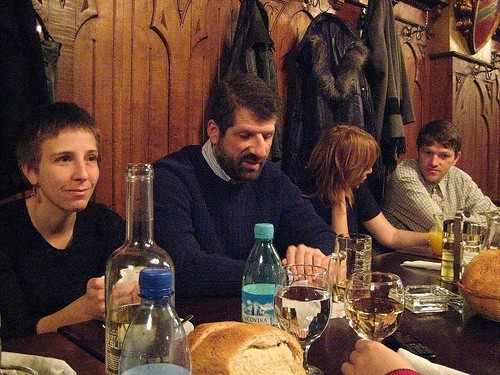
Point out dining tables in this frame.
[1,225,500,375]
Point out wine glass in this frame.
[343,271,405,344]
[274,264,333,375]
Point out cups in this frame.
[321,233,372,303]
[428,212,464,256]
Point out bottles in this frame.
[241,223,283,328]
[461,221,481,276]
[103,162,175,375]
[440,219,465,284]
[116,265,192,375]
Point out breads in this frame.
[176,320,305,375]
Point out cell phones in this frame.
[388,330,436,362]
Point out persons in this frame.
[0,100,149,334]
[382,119,499,234]
[140,73,344,307]
[296,125,430,255]
[340,339,422,375]
